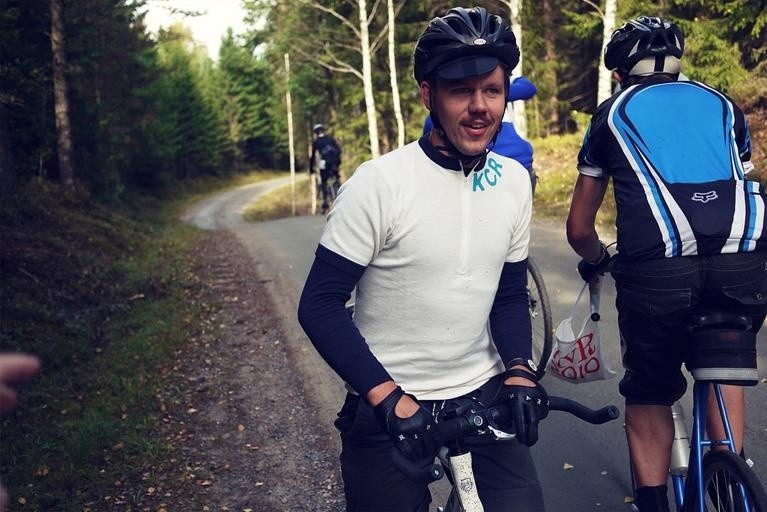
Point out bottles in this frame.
[670,402,690,475]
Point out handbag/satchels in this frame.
[544,312,616,384]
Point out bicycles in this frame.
[389,387,620,512]
[313,166,343,216]
[578,251,765,512]
[526,255,556,380]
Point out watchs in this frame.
[506,357,539,373]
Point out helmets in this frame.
[313,124,324,131]
[604,15,685,77]
[414,6,520,81]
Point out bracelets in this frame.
[588,240,608,267]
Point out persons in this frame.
[1,353,39,408]
[564,13,766,511]
[308,123,342,209]
[296,6,548,511]
[422,75,537,195]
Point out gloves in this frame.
[489,369,550,448]
[578,244,612,283]
[374,386,437,463]
